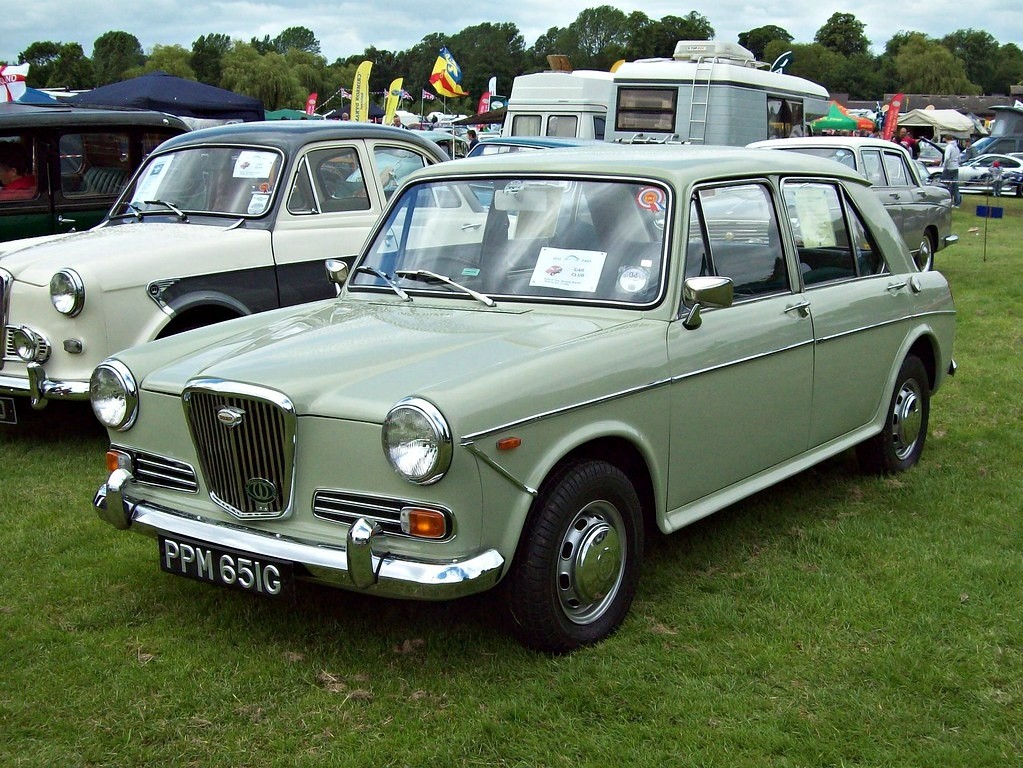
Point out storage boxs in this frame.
[976,206,1003,218]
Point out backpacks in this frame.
[909,142,920,160]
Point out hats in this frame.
[993,162,1000,167]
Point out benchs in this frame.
[611,240,875,301]
[70,165,129,196]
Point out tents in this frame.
[809,99,874,130]
[897,109,974,142]
[68,74,265,123]
[452,106,507,160]
[326,99,386,124]
[19,88,60,102]
[265,108,325,121]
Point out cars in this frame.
[90,144,956,655]
[332,111,502,199]
[656,136,958,272]
[926,154,1023,198]
[0,103,193,242]
[0,120,518,424]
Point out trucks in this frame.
[604,41,830,147]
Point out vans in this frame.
[952,137,1023,165]
[501,54,615,141]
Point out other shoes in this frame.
[951,204,960,208]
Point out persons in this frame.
[467,130,479,150]
[432,114,438,124]
[941,135,960,206]
[0,140,37,200]
[815,126,920,160]
[988,161,1003,197]
[393,115,409,131]
[965,139,976,160]
[342,113,349,121]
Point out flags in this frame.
[0,63,31,102]
[423,47,469,99]
[342,90,351,100]
[402,90,413,101]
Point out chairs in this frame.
[502,216,605,300]
